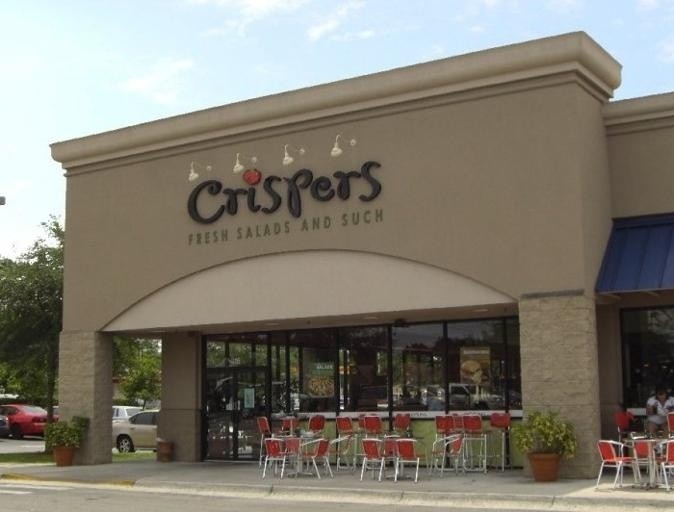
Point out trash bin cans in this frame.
[157,438,174,462]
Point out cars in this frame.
[0,414,9,437]
[0,404,59,439]
[111,406,144,422]
[44,405,59,416]
[110,409,160,454]
[449,383,511,409]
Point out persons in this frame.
[646,386,674,433]
[427,387,445,410]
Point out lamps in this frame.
[187,161,212,183]
[281,143,304,166]
[229,151,262,175]
[330,134,357,158]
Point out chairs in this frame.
[253,406,516,484]
[594,405,673,496]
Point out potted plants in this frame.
[42,415,91,466]
[512,412,580,483]
